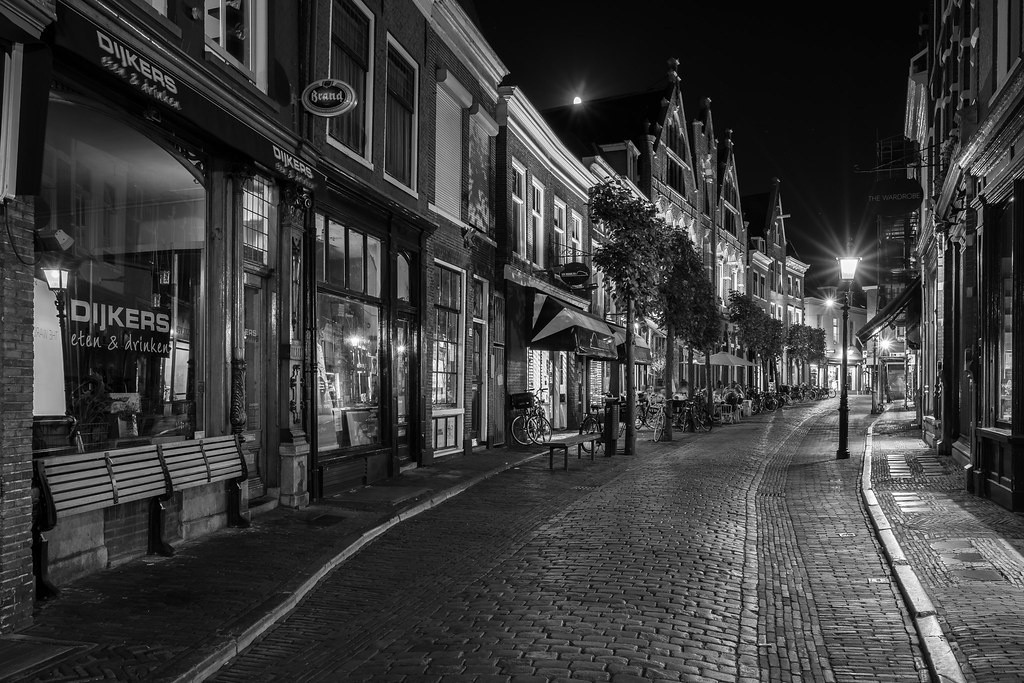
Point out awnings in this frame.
[533,293,652,365]
[855,273,922,352]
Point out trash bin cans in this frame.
[742,400,753,416]
[604,398,621,441]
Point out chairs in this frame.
[721,404,733,424]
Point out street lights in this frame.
[39,266,78,446]
[833,237,863,460]
[350,336,358,403]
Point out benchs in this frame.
[32,433,248,598]
[543,433,602,471]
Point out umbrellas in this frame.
[694,350,757,390]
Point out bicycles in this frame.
[511,388,552,445]
[745,385,836,415]
[579,392,614,453]
[635,389,714,443]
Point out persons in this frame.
[713,379,745,405]
